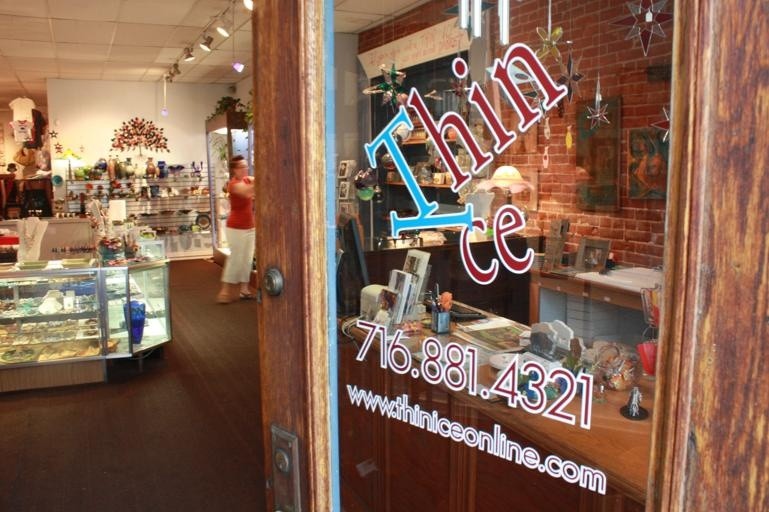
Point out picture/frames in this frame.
[574,95,620,214]
[627,126,667,201]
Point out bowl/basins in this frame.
[107,209,212,240]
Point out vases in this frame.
[158,161,166,177]
[125,158,134,179]
[120,162,127,179]
[146,157,155,175]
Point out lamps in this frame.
[162,76,169,117]
[165,0,254,87]
[229,1,244,74]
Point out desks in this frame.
[337,300,657,512]
[528,262,663,347]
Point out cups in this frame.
[26,208,77,220]
[122,300,146,345]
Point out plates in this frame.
[61,257,96,270]
[15,260,49,271]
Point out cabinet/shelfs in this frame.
[373,125,465,192]
[64,171,212,259]
[1,240,171,393]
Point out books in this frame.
[372,249,433,324]
[453,316,529,352]
[470,317,533,336]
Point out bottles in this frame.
[402,109,427,141]
[84,154,167,180]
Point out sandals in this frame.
[240,291,257,300]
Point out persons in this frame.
[214,155,257,303]
